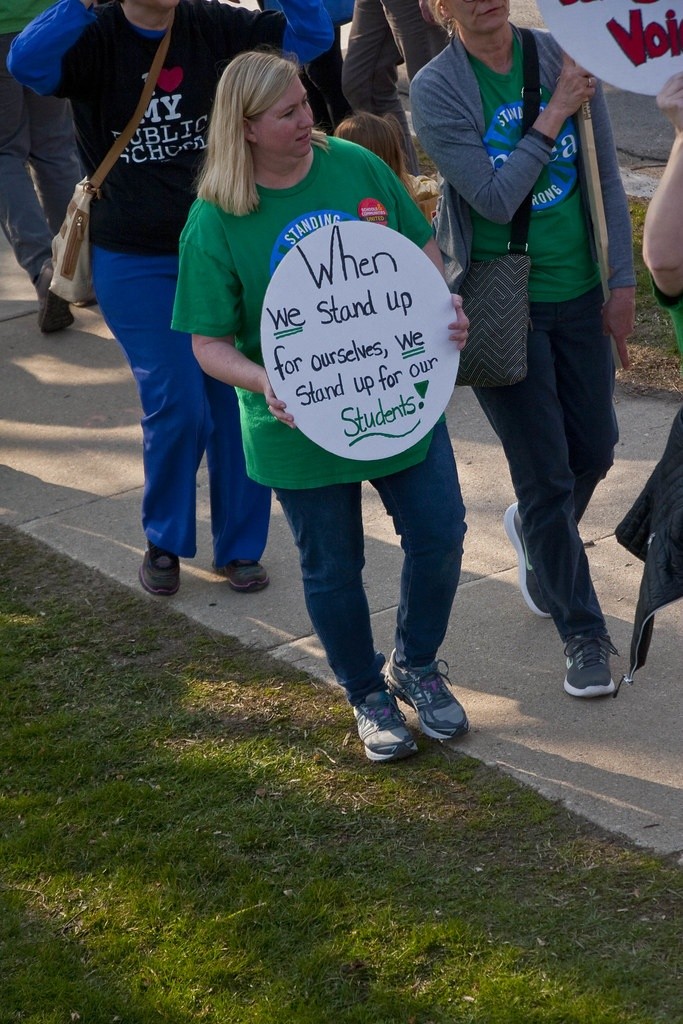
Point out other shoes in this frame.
[75,292,97,307]
[33,260,75,334]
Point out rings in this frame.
[267,406,271,412]
[274,416,278,421]
[588,77,591,87]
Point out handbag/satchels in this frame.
[48,176,101,304]
[454,254,531,385]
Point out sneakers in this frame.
[504,501,552,618]
[138,538,179,596]
[216,559,270,593]
[353,686,418,762]
[384,647,470,740]
[563,631,620,697]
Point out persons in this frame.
[615,71,683,686]
[259,0,453,228]
[0,0,335,600]
[170,54,471,759]
[409,0,638,696]
